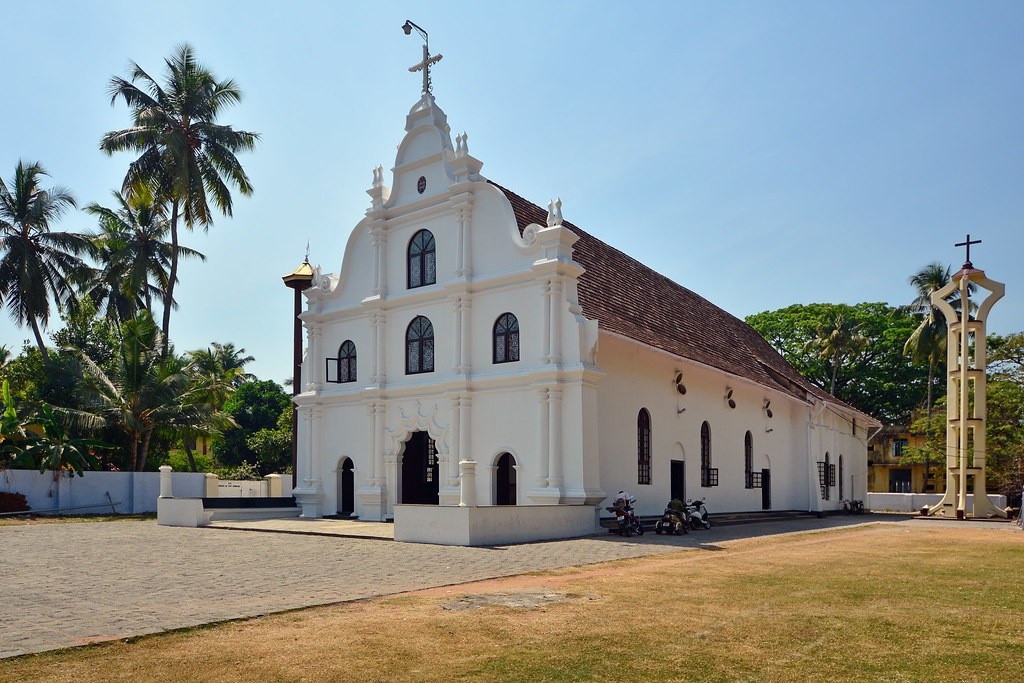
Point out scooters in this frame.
[687,496,713,530]
[655,510,689,534]
[606,500,642,537]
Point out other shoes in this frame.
[606,506,614,513]
[618,533,623,536]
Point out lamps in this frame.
[677,408,687,417]
[765,429,774,434]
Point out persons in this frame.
[668,497,683,510]
[614,490,634,511]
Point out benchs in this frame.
[191,496,303,524]
